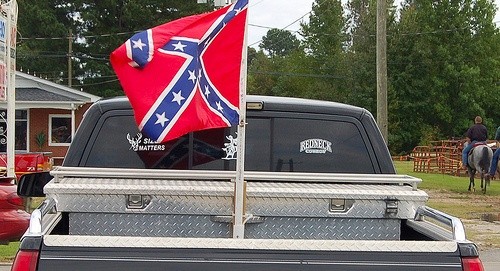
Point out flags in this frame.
[110,0,249,143]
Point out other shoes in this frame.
[459,166,466,170]
[489,173,494,178]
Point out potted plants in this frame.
[398,151,410,160]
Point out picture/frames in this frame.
[48,114,75,146]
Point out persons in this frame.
[461,116,500,178]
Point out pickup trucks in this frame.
[12,92,484,271]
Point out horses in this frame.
[463,136,493,194]
